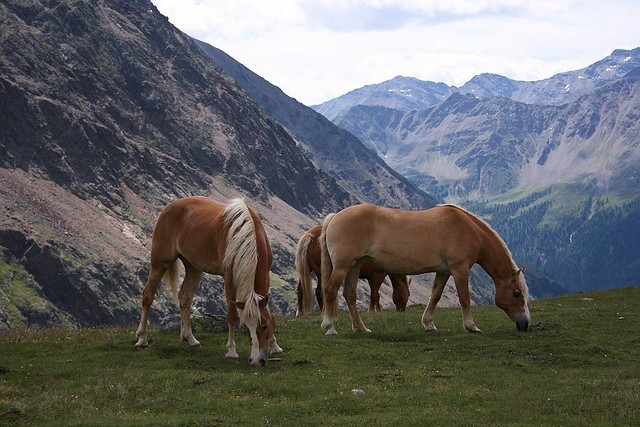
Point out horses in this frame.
[292,223,412,320]
[135,195,284,368]
[318,203,532,337]
